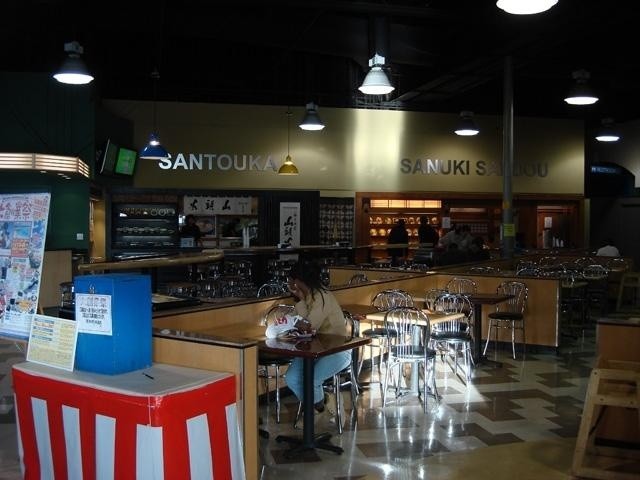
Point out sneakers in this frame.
[296,405,327,429]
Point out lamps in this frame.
[276,106,299,175]
[357,49,396,97]
[299,97,327,132]
[494,0,561,17]
[51,41,96,85]
[137,101,171,161]
[562,64,599,108]
[451,102,482,138]
[593,105,623,143]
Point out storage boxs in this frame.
[70,272,158,377]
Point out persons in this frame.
[469,236,491,261]
[417,216,440,246]
[596,238,620,258]
[221,218,241,237]
[440,225,474,249]
[386,219,408,265]
[180,213,201,282]
[282,261,351,429]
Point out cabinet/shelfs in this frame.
[368,209,440,259]
[10,355,247,480]
[109,202,178,255]
